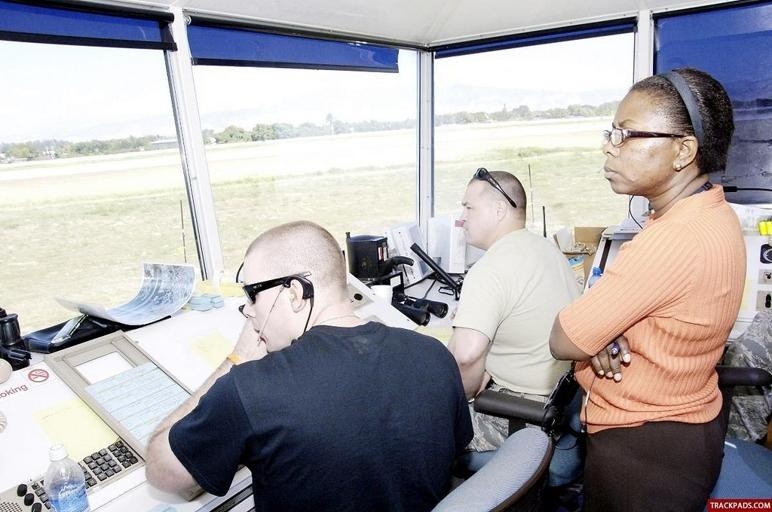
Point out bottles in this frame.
[43,443,91,511]
[588,267,603,289]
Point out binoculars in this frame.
[391,293,451,327]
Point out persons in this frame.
[549,67,748,511]
[443,168,583,454]
[144,219,477,512]
[725,309,772,449]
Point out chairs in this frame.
[705,366,771,512]
[428,360,581,511]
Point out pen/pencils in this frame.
[88,318,108,328]
[611,343,620,356]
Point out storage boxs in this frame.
[553,226,606,291]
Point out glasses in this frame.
[243,272,311,305]
[600,129,684,146]
[475,168,517,208]
